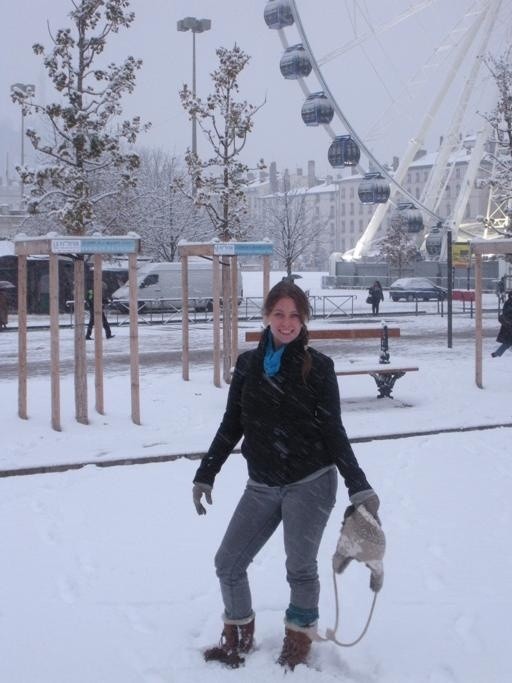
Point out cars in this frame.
[388,276,448,303]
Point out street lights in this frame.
[175,14,212,197]
[434,219,455,349]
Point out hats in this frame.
[330,502,387,594]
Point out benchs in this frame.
[243,325,419,400]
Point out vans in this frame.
[104,260,245,316]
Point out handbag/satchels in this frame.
[365,295,373,304]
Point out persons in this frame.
[369,280,384,315]
[85,284,117,340]
[489,289,511,357]
[190,281,382,669]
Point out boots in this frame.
[276,617,320,672]
[202,611,259,669]
